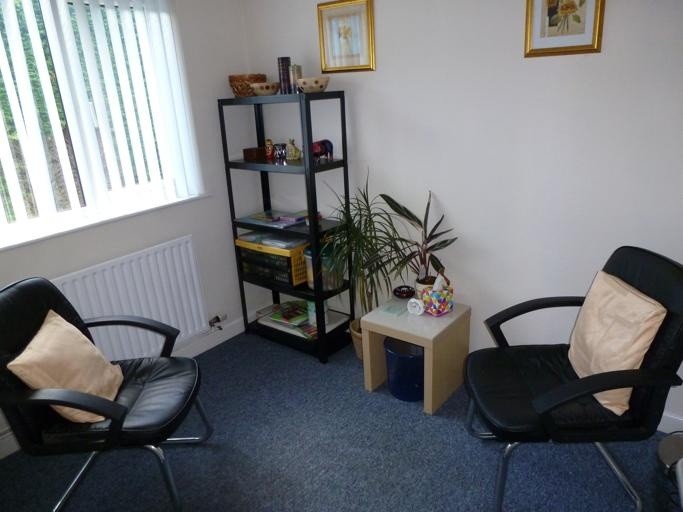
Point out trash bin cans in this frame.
[383,336,424,402]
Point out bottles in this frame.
[288,64,302,94]
[277,57,290,95]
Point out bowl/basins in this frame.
[296,77,330,93]
[228,72,268,98]
[249,82,279,96]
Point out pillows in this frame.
[6,309,123,422]
[560,270,666,417]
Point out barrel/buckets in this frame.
[303,242,345,290]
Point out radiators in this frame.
[48,234,211,362]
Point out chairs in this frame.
[0,277,213,512]
[461,246,683,512]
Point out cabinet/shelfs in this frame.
[218,91,355,364]
[360,299,471,415]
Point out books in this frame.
[235,231,307,288]
[255,299,317,339]
[233,208,321,230]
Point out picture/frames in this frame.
[317,0,375,74]
[525,0,606,57]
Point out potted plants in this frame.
[315,166,420,362]
[360,191,457,300]
